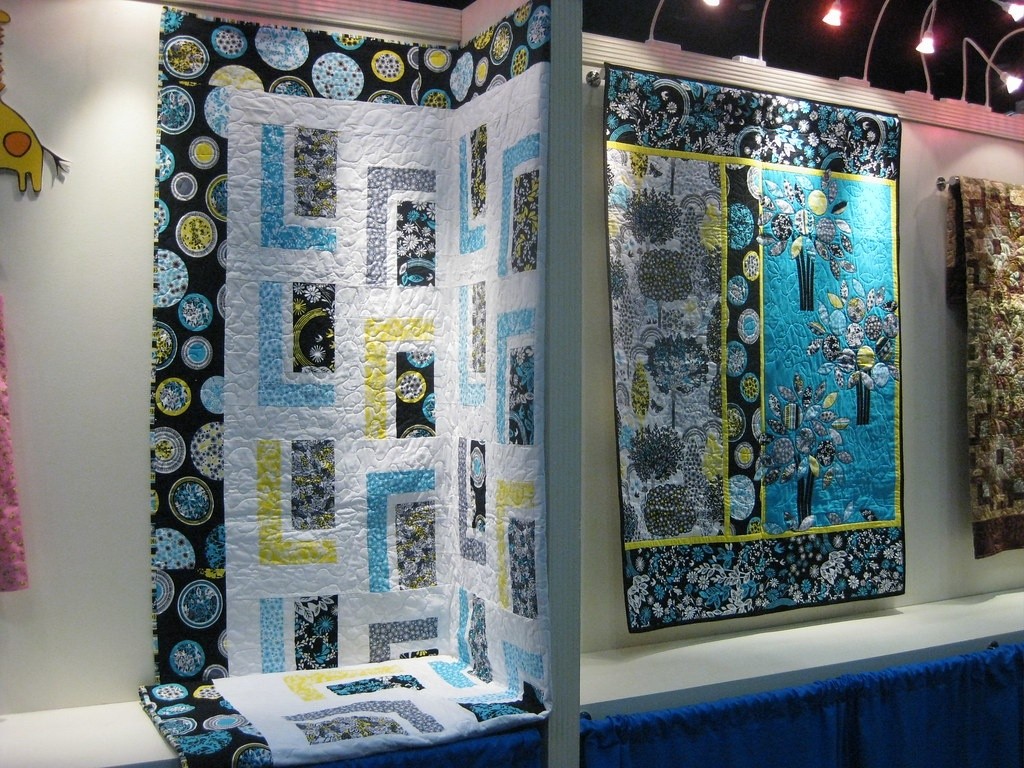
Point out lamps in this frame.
[650,0,1024,107]
